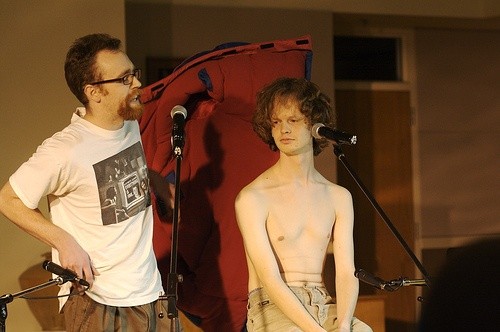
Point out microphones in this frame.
[311,123,358,145]
[354,267,394,292]
[43,260,89,286]
[170,105,187,156]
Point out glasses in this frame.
[88,68,142,85]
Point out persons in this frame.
[0,31,206,332]
[234,77,374,332]
